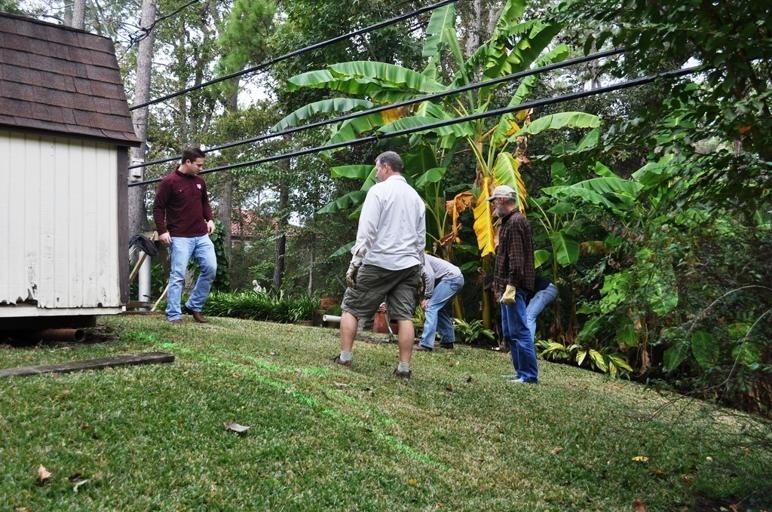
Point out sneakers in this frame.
[393,368,411,380]
[330,354,352,365]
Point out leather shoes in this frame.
[502,374,536,384]
[414,345,432,351]
[172,319,182,323]
[185,305,208,322]
[434,342,453,348]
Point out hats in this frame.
[486,186,517,202]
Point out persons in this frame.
[413,252,465,352]
[486,185,539,384]
[329,150,426,380]
[152,148,218,323]
[484,275,560,359]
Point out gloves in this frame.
[346,263,357,288]
[500,284,516,304]
